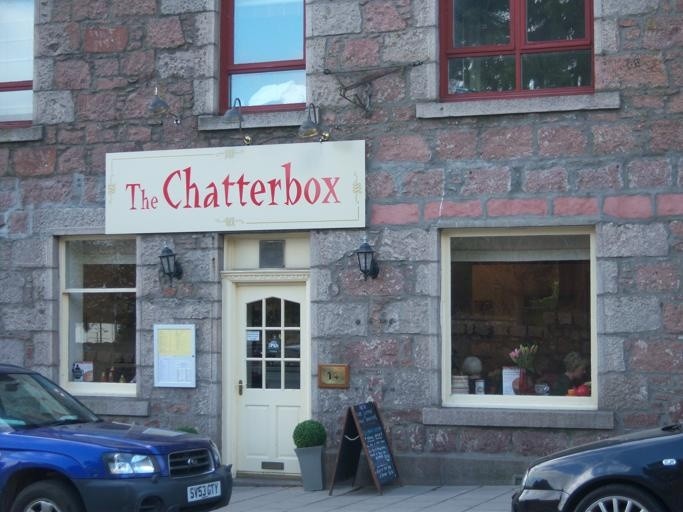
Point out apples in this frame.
[575,384,590,396]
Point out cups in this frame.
[534,383,551,394]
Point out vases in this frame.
[512,369,533,395]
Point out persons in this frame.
[460,356,498,394]
[550,352,589,395]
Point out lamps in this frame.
[160,247,182,280]
[354,243,379,279]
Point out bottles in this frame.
[100,365,127,383]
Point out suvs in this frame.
[0,361,231,511]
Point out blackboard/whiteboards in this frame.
[351,401,401,487]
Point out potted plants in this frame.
[292,419,327,492]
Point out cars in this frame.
[510,422,682,511]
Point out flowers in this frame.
[509,345,541,375]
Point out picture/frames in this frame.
[153,324,196,389]
[318,364,350,389]
